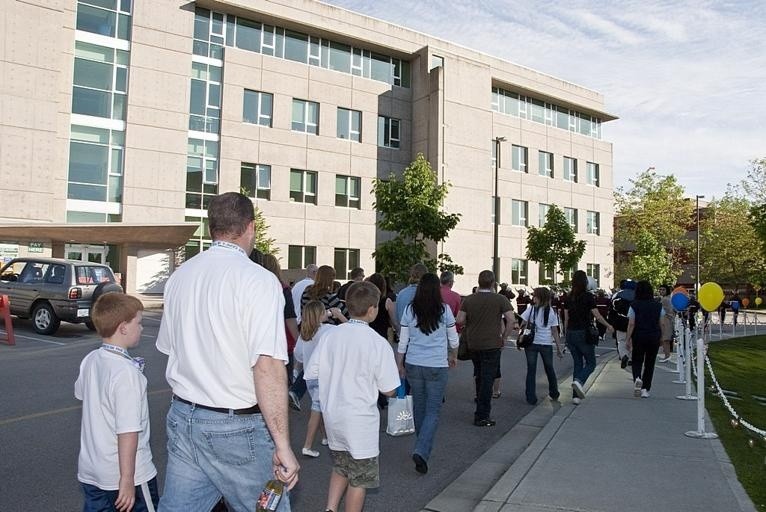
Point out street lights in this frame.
[696,195,705,302]
[492,136,508,295]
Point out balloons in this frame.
[671,293,689,311]
[698,282,725,311]
[742,298,750,308]
[755,297,762,306]
[672,287,688,294]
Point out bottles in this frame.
[325,309,341,317]
[256,478,283,511]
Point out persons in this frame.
[550,289,612,341]
[397,272,460,473]
[457,270,516,427]
[678,293,742,331]
[303,282,401,511]
[471,283,532,401]
[625,281,666,398]
[517,287,562,404]
[251,250,462,456]
[73,290,160,511]
[563,271,615,404]
[155,192,301,512]
[610,279,673,368]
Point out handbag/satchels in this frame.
[517,321,535,346]
[386,395,415,436]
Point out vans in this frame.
[686,288,696,299]
[0,257,124,335]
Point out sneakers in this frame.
[288,390,301,410]
[621,354,671,397]
[302,447,319,457]
[475,416,495,426]
[413,453,428,473]
[572,380,585,405]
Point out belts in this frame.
[173,394,260,415]
